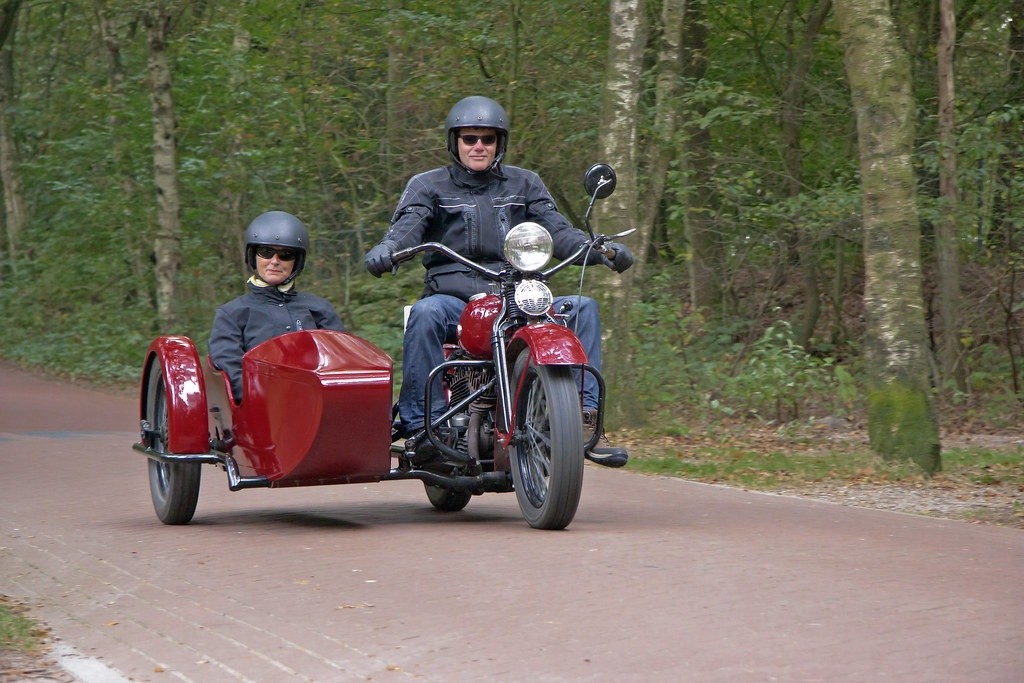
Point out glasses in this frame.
[255,246,297,262]
[459,134,496,145]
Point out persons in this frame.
[208,211,353,401]
[366,96,634,468]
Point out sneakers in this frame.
[407,434,439,465]
[583,409,628,468]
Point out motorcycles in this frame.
[132,160,636,529]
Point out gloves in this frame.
[589,242,633,273]
[364,239,399,279]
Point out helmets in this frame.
[245,210,309,275]
[447,95,510,152]
